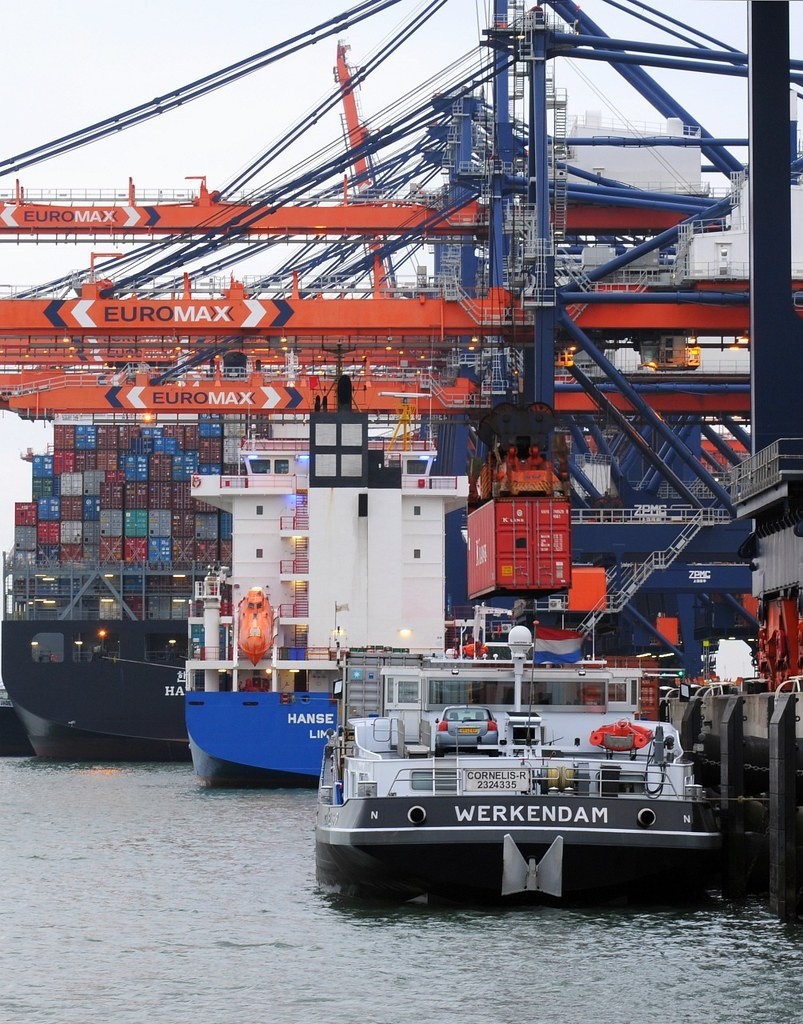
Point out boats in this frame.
[184,412,336,782]
[309,619,721,913]
[1,536,231,744]
[238,586,274,667]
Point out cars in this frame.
[434,703,499,758]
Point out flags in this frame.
[534,627,583,665]
[336,604,349,612]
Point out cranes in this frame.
[0,0,802,451]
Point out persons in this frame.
[527,446,543,469]
[504,447,519,471]
